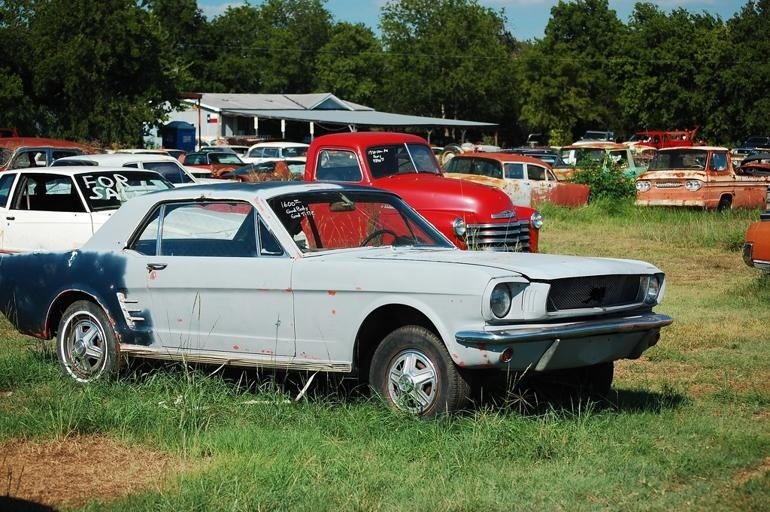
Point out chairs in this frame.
[259,222,295,256]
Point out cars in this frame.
[741,213,770,270]
[0,135,331,260]
[426,130,710,210]
[0,179,678,425]
[729,136,770,192]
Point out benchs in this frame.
[136,239,255,257]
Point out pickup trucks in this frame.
[633,145,770,213]
[200,131,545,256]
